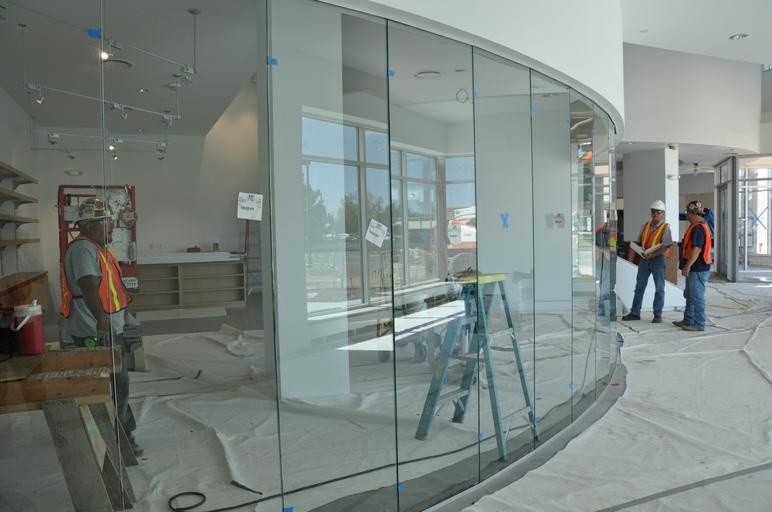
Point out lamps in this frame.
[0,1,196,158]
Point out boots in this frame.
[426,224,476,274]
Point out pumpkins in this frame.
[414,273,540,462]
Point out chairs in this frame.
[652,317,662,323]
[672,320,704,331]
[622,314,641,321]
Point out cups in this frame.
[650,209,663,215]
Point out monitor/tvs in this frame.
[628,240,676,259]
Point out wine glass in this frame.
[684,272,686,273]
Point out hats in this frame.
[325,232,401,243]
[595,222,622,247]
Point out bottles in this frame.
[684,200,707,215]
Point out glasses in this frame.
[0,1,196,158]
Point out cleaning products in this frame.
[375,300,489,361]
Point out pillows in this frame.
[72,197,113,222]
[647,199,666,212]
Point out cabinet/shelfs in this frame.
[0,160,247,347]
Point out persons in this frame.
[56,196,145,457]
[620,199,675,323]
[670,200,712,331]
[593,203,618,317]
